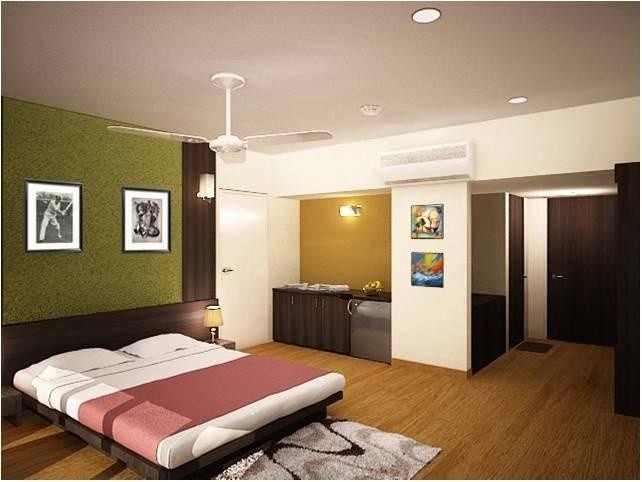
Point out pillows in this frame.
[120,333,208,359]
[26,347,135,381]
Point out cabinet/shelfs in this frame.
[298,292,351,356]
[272,288,298,346]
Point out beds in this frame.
[1,298,345,479]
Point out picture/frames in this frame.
[121,186,172,253]
[25,180,83,253]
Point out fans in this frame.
[106,73,333,164]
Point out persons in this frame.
[38,195,67,242]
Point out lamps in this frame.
[204,305,223,345]
[196,173,215,203]
[359,104,383,117]
[338,204,362,216]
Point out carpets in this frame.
[515,340,554,354]
[213,417,442,480]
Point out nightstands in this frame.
[1,385,23,427]
[204,338,235,351]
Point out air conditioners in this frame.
[380,142,474,186]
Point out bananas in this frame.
[364,279,382,294]
